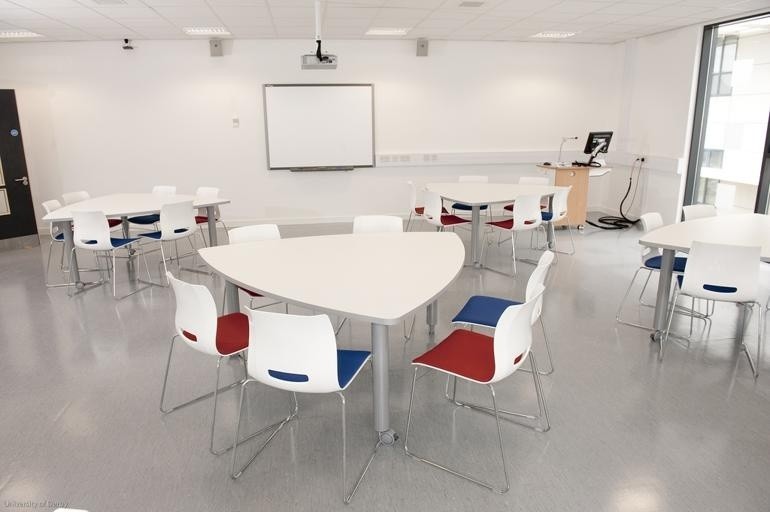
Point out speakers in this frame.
[416,38,428,57]
[210,39,222,56]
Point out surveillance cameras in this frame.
[123,38,131,45]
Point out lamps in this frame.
[555,136,578,168]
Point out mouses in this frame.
[543,161,550,165]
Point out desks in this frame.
[635,211,770,348]
[535,161,626,228]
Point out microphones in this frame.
[557,136,578,165]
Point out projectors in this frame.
[300,54,337,70]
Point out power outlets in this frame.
[633,157,645,164]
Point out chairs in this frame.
[335,214,420,345]
[41,181,235,302]
[226,302,388,504]
[443,248,556,423]
[219,221,297,331]
[613,212,714,342]
[681,202,720,223]
[398,177,580,279]
[401,281,551,496]
[156,269,303,457]
[656,239,768,380]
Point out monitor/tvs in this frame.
[584,131,614,154]
